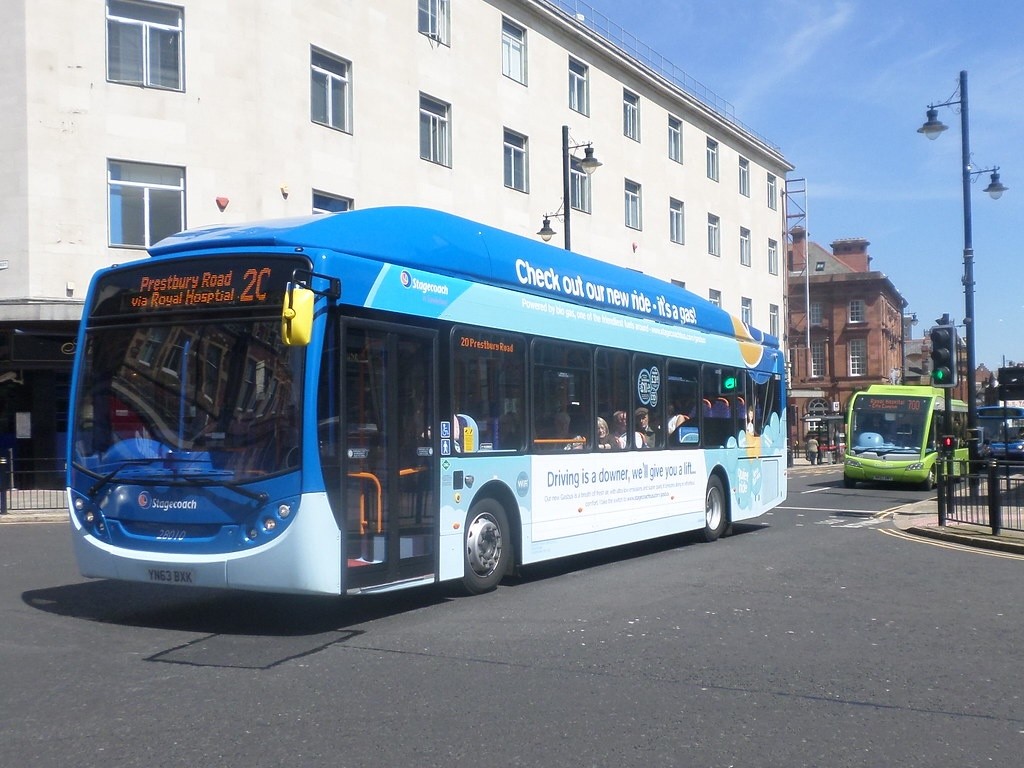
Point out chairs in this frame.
[690,395,746,417]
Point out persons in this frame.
[635,408,662,450]
[808,436,819,465]
[667,395,685,436]
[613,410,643,450]
[595,416,621,450]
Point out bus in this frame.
[976,407,1024,461]
[64,204,787,598]
[843,385,970,491]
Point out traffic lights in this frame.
[929,326,958,388]
[942,434,955,452]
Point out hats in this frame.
[636,408,648,417]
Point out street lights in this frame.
[536,124,604,253]
[915,69,1011,505]
